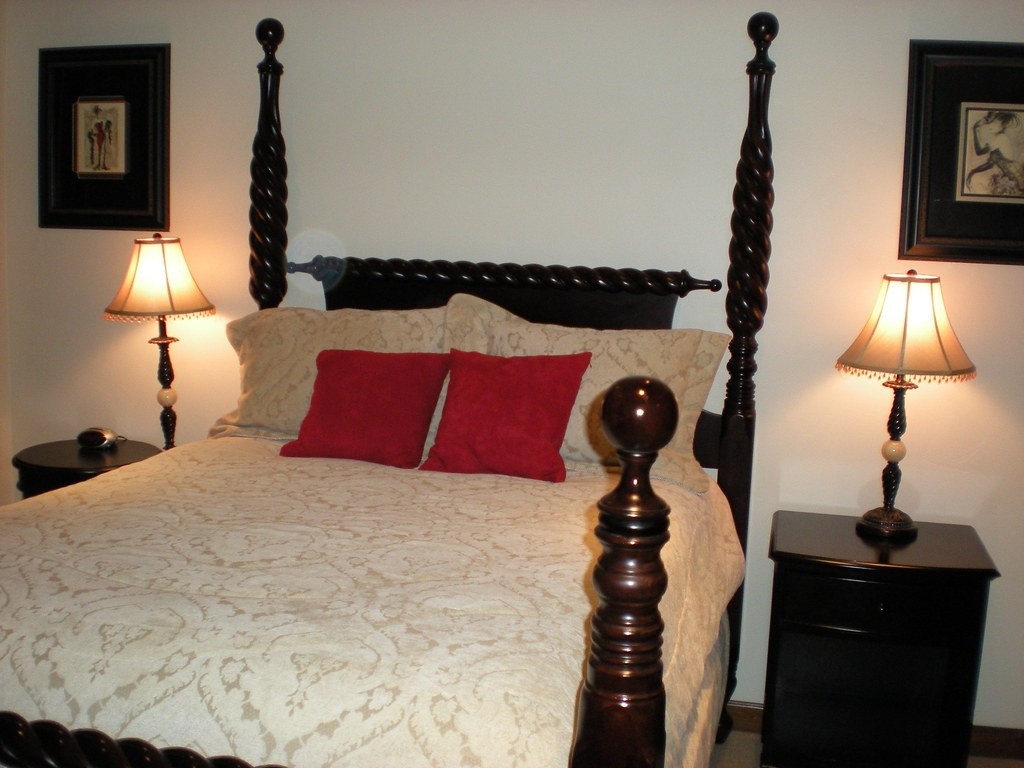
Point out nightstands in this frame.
[761,510,1001,768]
[12,436,162,499]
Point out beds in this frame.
[0,13,779,768]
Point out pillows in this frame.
[211,292,733,491]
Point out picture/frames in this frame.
[898,39,1024,266]
[37,43,171,232]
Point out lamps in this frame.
[104,234,216,450]
[832,269,978,548]
